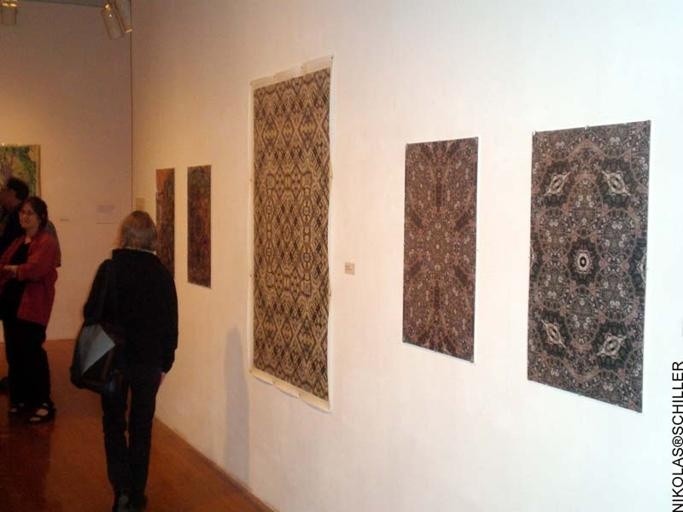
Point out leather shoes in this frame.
[112,488,147,511]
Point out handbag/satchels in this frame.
[70,318,118,394]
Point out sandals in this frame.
[8,402,55,422]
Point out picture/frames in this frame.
[245,54,334,414]
[0,144,40,198]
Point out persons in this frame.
[0,177,62,424]
[83,210,178,512]
